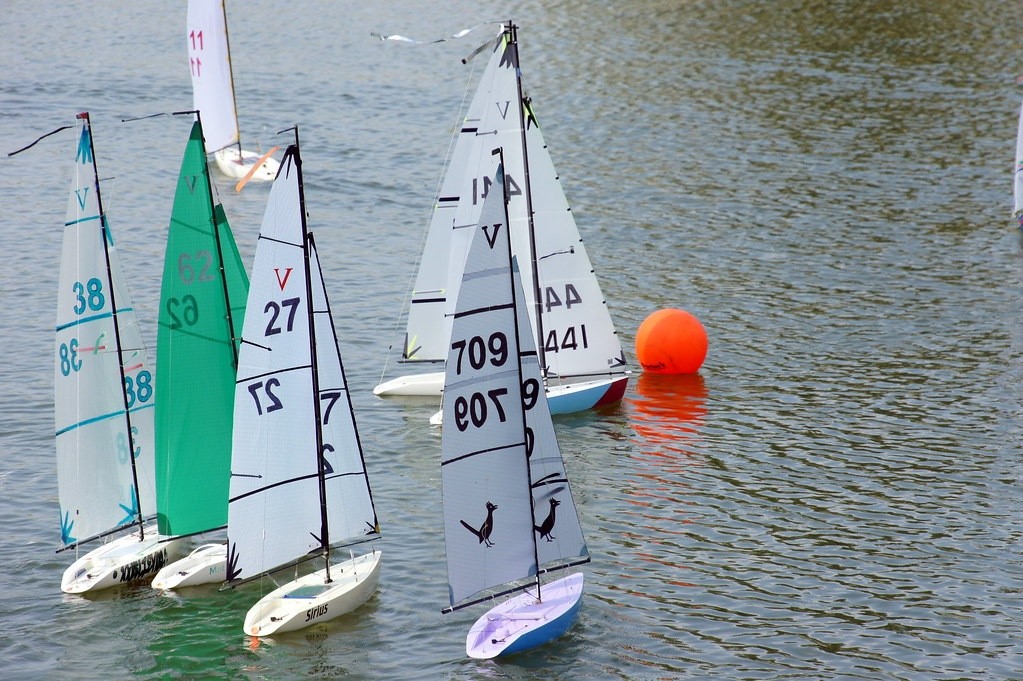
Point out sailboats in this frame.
[438,147,592,659]
[52,112,251,594]
[225,125,383,636]
[374,19,629,426]
[187,1,281,183]
